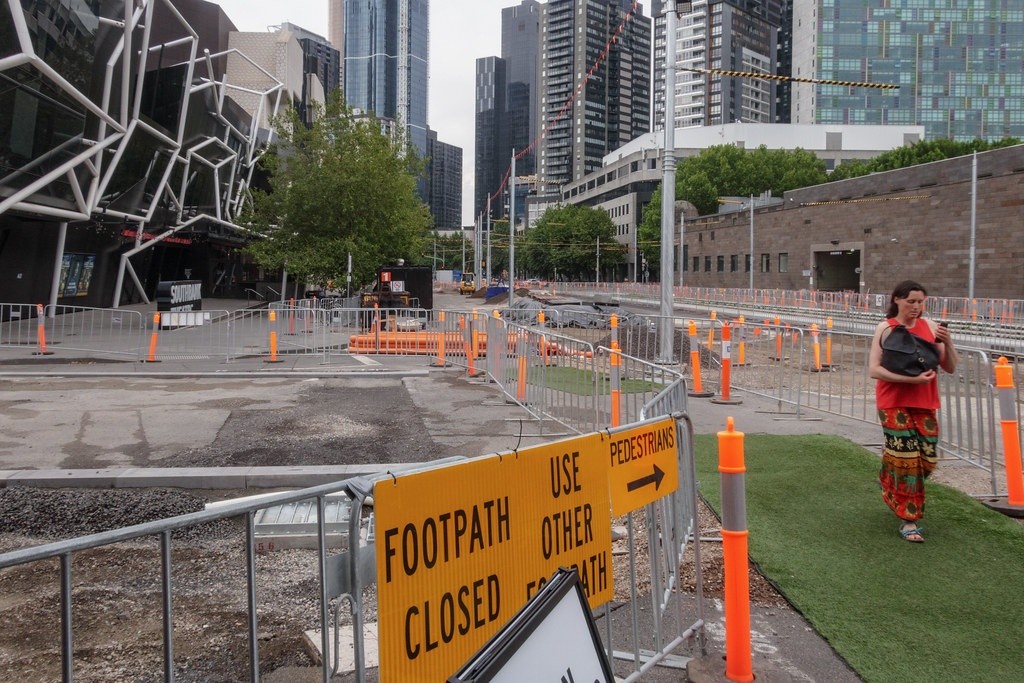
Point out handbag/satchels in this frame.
[878,324,940,377]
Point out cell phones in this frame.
[935,322,948,343]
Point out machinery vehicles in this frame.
[461,272,477,294]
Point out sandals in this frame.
[898,520,924,542]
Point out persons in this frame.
[868,280,957,542]
